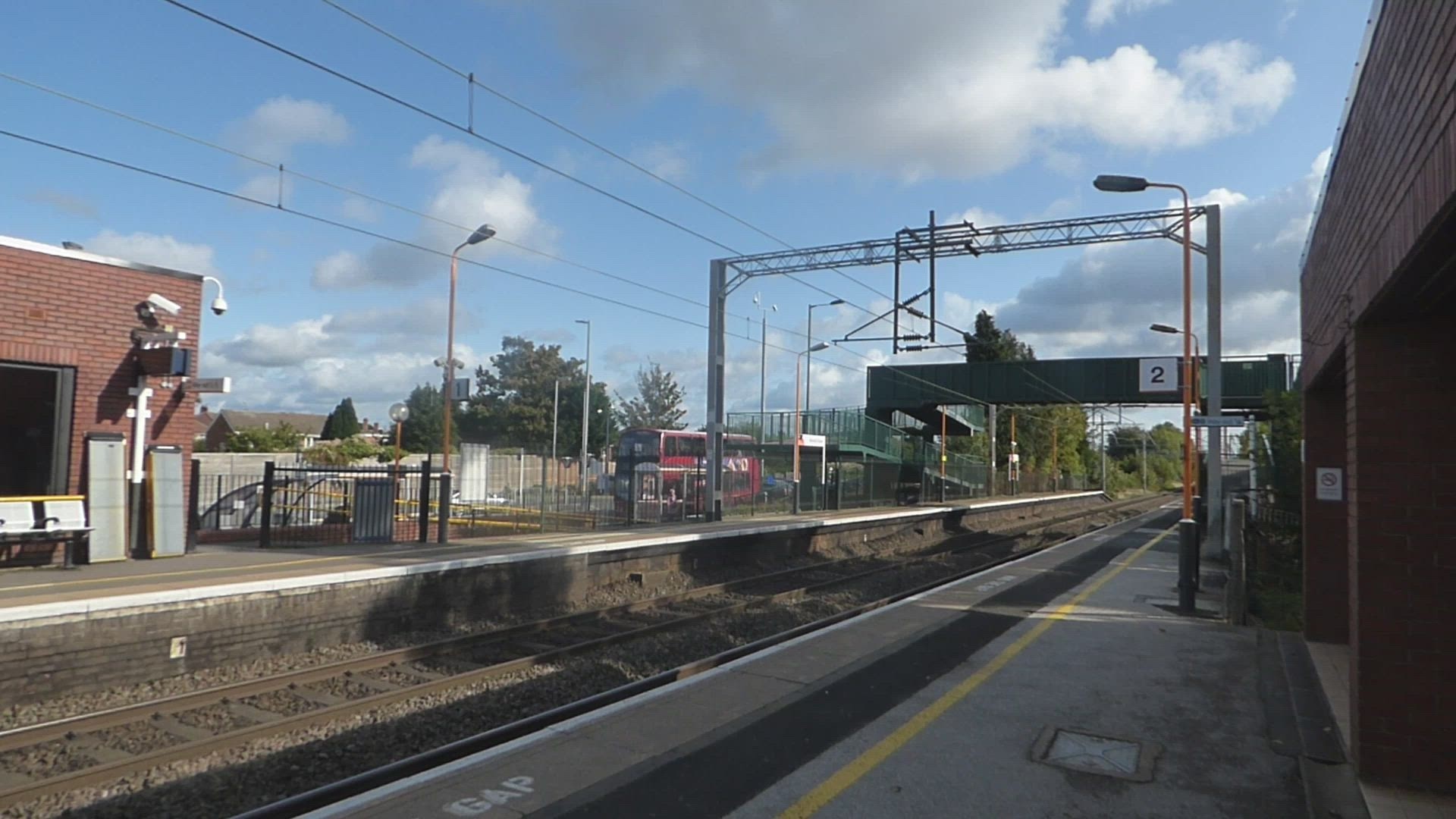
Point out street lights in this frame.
[803,298,848,430]
[575,318,592,503]
[789,342,828,512]
[1092,173,1199,615]
[431,223,499,542]
[1150,322,1201,524]
[388,403,410,505]
[751,292,777,498]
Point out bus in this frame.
[608,426,761,516]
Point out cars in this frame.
[758,480,794,498]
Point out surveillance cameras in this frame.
[211,299,227,315]
[146,293,181,315]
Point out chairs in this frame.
[0,493,98,570]
